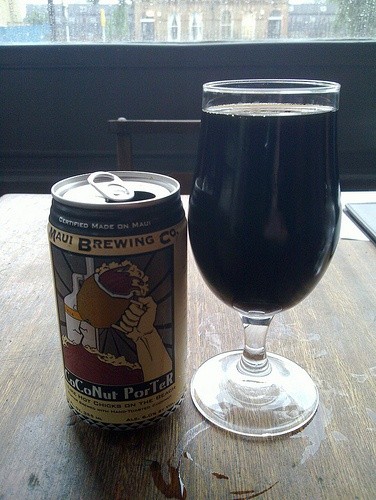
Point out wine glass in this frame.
[185,77,340,439]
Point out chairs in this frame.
[108,116,200,196]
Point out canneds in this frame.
[48,171,187,429]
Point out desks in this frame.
[0,192,376,500]
